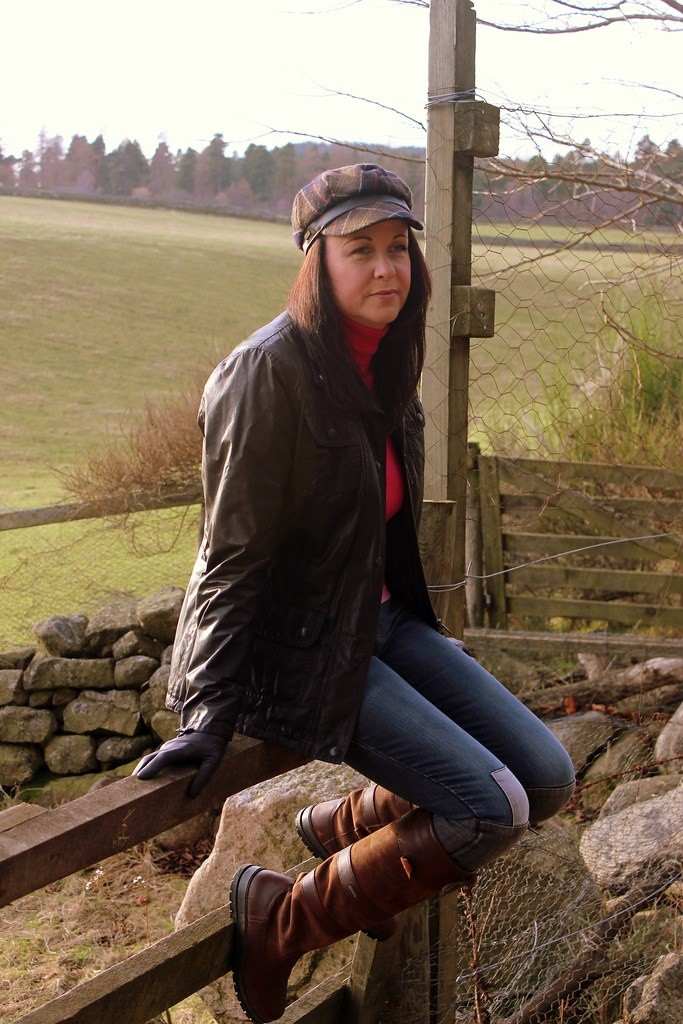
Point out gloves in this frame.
[131,725,229,801]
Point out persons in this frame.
[131,162,577,1024]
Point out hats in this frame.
[292,163,423,255]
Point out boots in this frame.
[229,807,479,1024]
[294,783,418,941]
